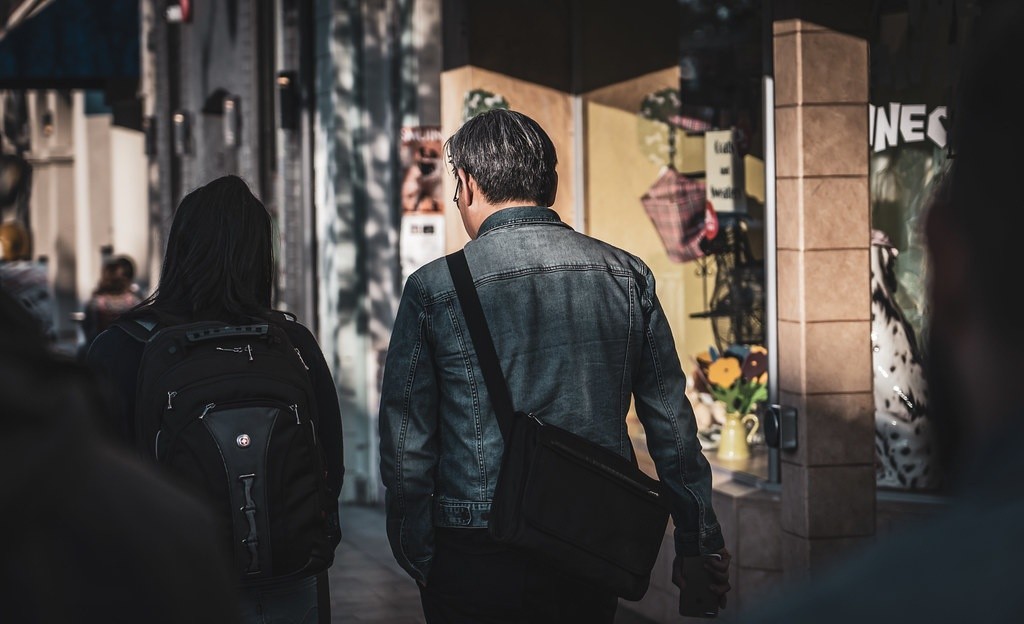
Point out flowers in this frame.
[692,343,769,415]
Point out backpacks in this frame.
[115,307,340,589]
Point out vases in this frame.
[717,411,759,462]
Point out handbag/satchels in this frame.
[487,412,674,600]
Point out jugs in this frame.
[716,411,759,462]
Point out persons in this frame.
[1,222,29,261]
[86,257,142,343]
[0,262,244,624]
[86,175,345,624]
[380,106,731,624]
